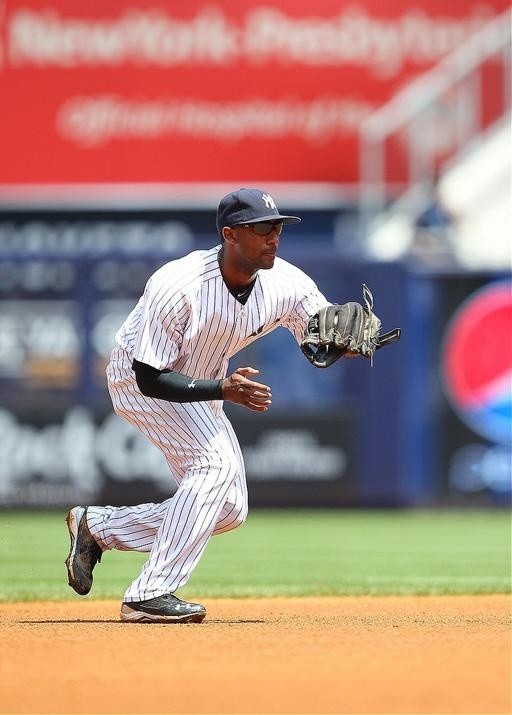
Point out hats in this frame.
[217,189,302,233]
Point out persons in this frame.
[66,189,401,622]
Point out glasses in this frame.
[234,223,285,237]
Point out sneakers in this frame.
[120,593,208,624]
[64,505,101,595]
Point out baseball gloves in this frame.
[300,300,404,368]
